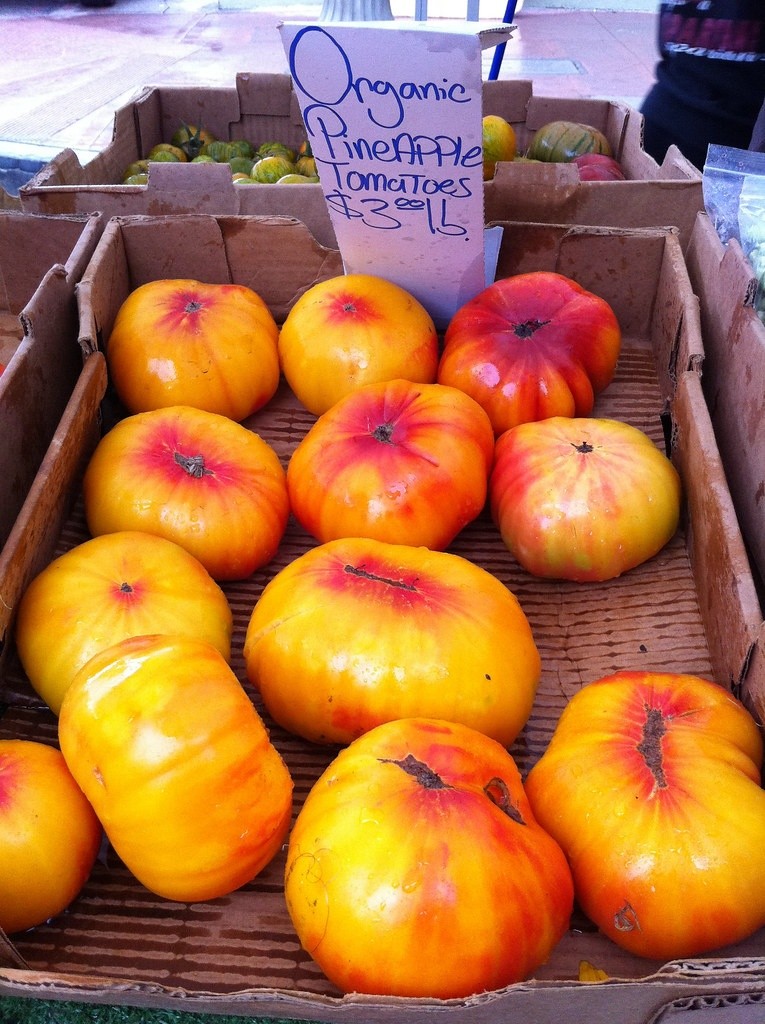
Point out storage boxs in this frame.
[1,75,765,1024]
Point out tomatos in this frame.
[0,271,765,998]
[122,115,626,186]
